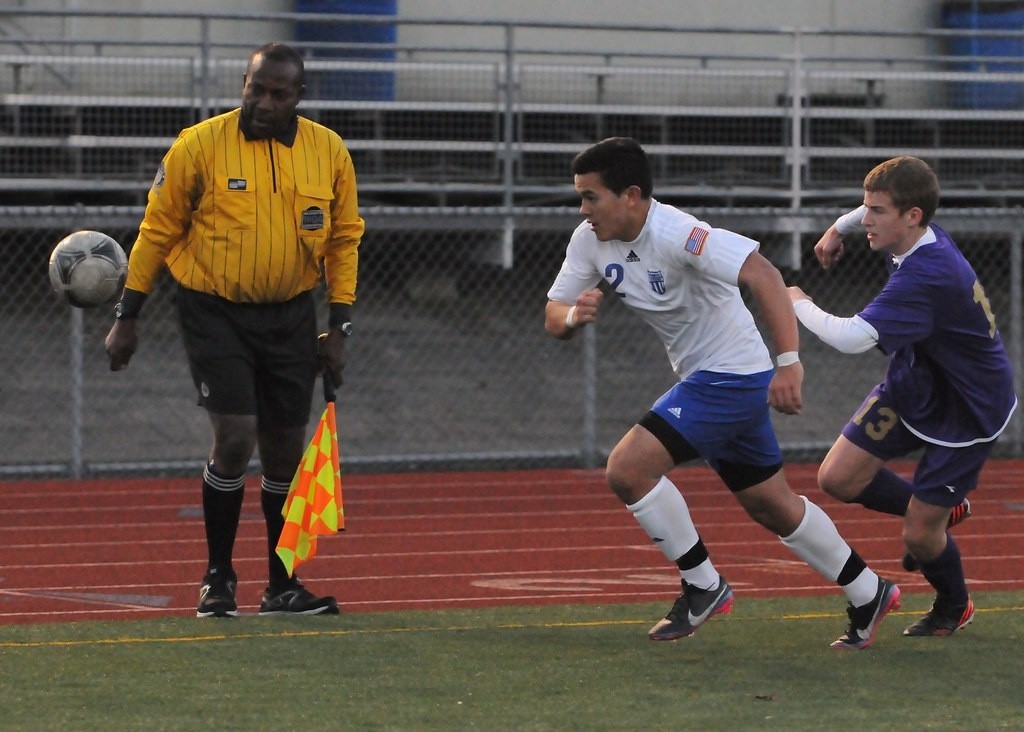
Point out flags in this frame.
[275,401,346,580]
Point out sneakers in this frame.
[648,575,735,641]
[830,576,901,650]
[258,574,340,616]
[903,594,975,636]
[902,497,972,572]
[196,566,237,617]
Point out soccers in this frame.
[47,229,130,310]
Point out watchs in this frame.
[112,300,138,320]
[335,322,353,335]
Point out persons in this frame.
[786,156,1019,636]
[542,135,900,652]
[104,41,366,617]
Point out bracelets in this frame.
[565,306,576,329]
[776,351,801,367]
